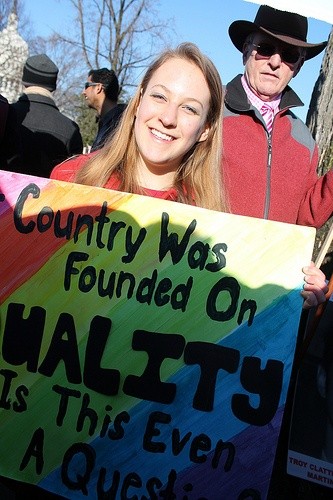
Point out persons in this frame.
[222,3,333,231]
[49,40,329,313]
[83,68,128,153]
[0,53,83,179]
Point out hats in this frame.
[21,53,59,91]
[228,5,328,61]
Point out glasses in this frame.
[84,82,101,90]
[250,39,301,68]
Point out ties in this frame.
[260,103,275,132]
